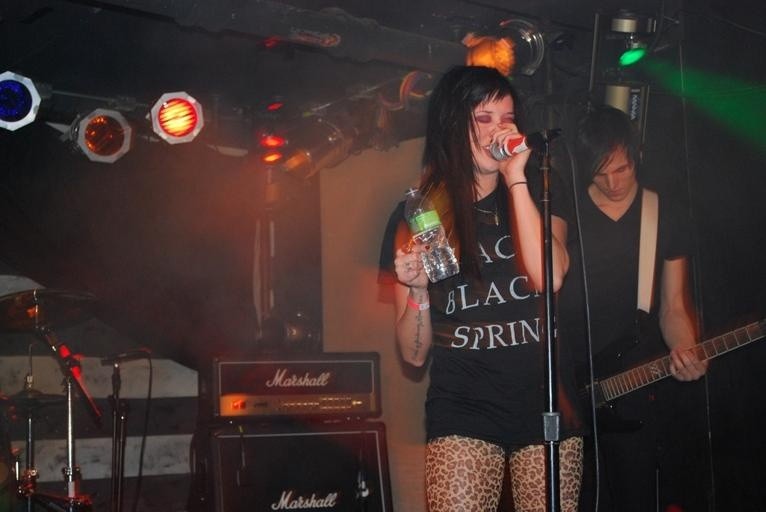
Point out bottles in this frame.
[402,184,462,285]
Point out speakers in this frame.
[194,419,393,511]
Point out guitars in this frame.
[554,322,765,440]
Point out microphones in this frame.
[100,344,149,368]
[490,127,550,159]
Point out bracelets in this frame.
[507,181,528,194]
[406,289,431,311]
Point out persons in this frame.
[540,102,720,512]
[377,63,596,511]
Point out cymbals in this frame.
[1,389,64,404]
[1,290,100,333]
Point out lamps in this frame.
[62,108,135,168]
[144,90,204,146]
[459,20,546,83]
[0,69,42,131]
[595,79,652,168]
[282,114,358,180]
[255,112,293,152]
[608,11,655,68]
